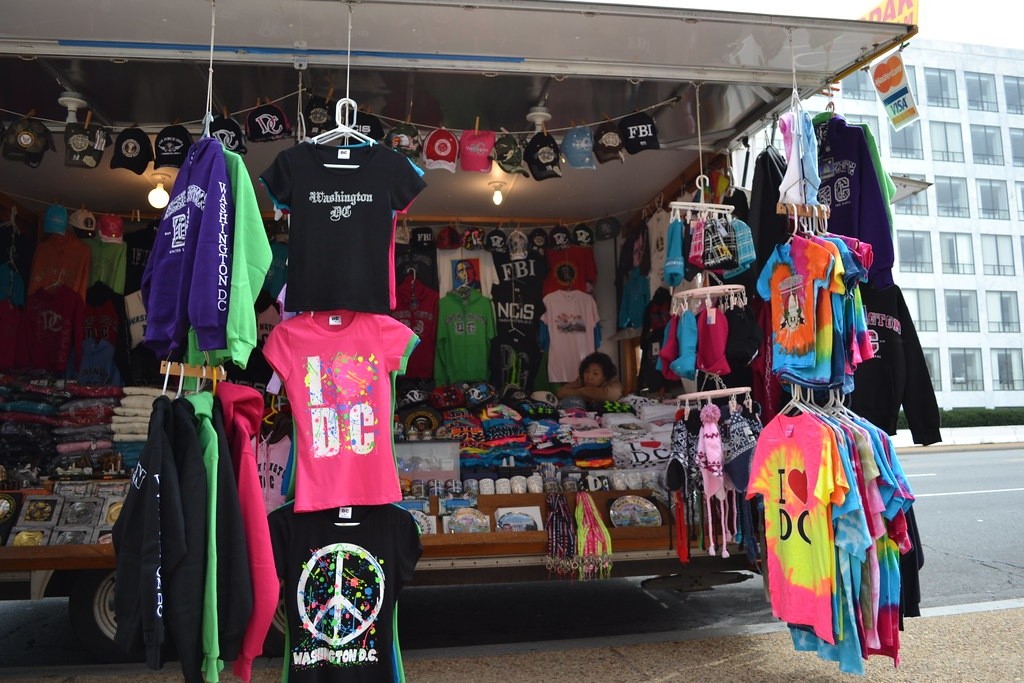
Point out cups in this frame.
[400,471,658,499]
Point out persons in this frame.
[633,369,685,399]
[556,350,623,403]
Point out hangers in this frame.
[407,267,416,289]
[508,316,525,336]
[507,261,525,283]
[86,276,108,296]
[7,281,16,306]
[7,245,19,272]
[641,206,651,222]
[655,192,664,214]
[161,362,226,399]
[198,112,215,139]
[45,269,65,290]
[455,270,470,291]
[311,97,375,144]
[262,388,285,425]
[778,380,860,428]
[784,202,832,245]
[725,167,738,197]
[561,275,574,292]
[825,102,836,120]
[85,323,102,344]
[0,204,21,233]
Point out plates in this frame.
[56,532,86,544]
[107,503,123,525]
[408,509,432,535]
[13,532,43,546]
[610,495,662,528]
[67,502,96,524]
[25,501,51,520]
[448,508,489,533]
[0,494,16,524]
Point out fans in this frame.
[57,87,87,122]
[525,105,554,131]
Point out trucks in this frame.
[0,0,933,666]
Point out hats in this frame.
[460,131,495,171]
[340,113,382,147]
[654,219,761,378]
[385,125,421,162]
[394,218,619,260]
[303,98,339,140]
[594,123,626,165]
[421,129,460,173]
[3,117,245,174]
[619,113,658,155]
[246,107,291,142]
[561,129,596,170]
[44,204,125,242]
[488,136,530,177]
[265,217,290,242]
[524,132,563,181]
[662,401,765,564]
